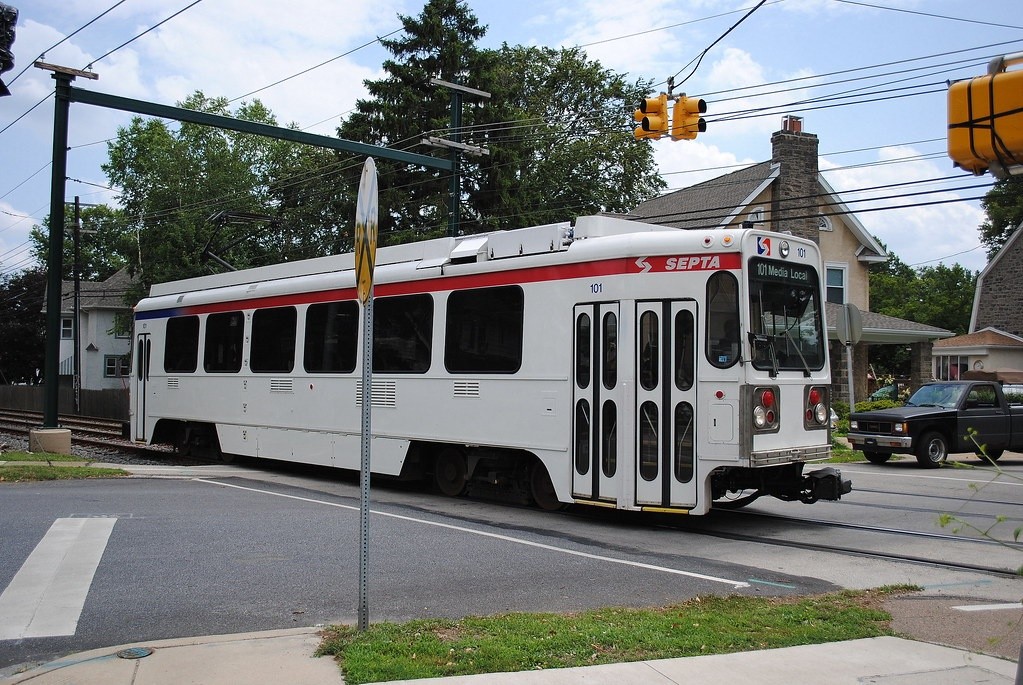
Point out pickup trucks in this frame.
[847,380,1023,470]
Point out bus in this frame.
[121,215,852,518]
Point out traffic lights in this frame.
[634,109,661,140]
[678,96,706,139]
[640,95,668,133]
